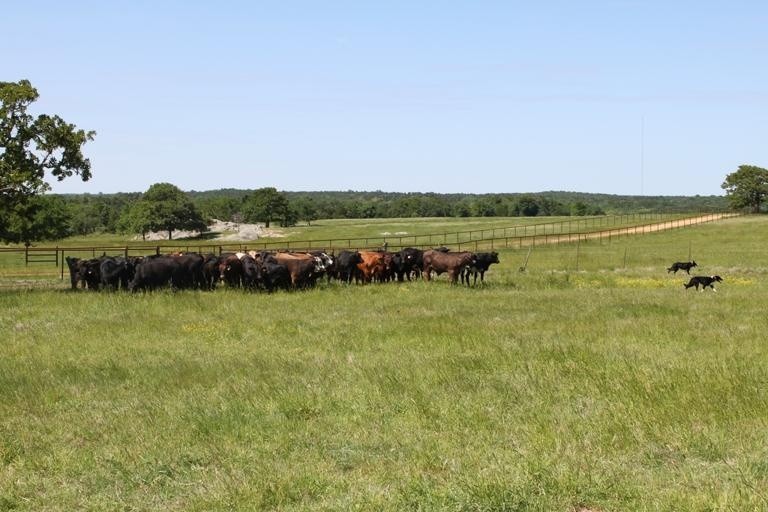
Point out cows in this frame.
[63,246,422,294]
[461,251,500,289]
[422,250,478,287]
[420,246,450,254]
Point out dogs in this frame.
[683,275,723,292]
[666,260,698,274]
